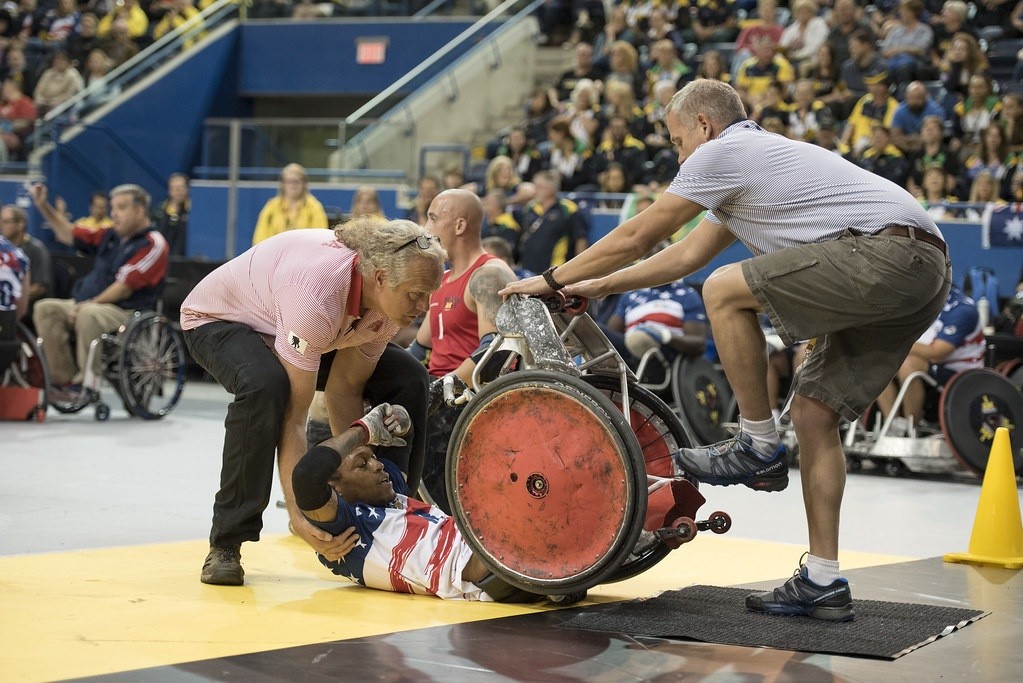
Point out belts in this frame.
[871,225,946,254]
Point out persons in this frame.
[181,218,447,584]
[497,80,954,621]
[0,0,1023,462]
[294,405,542,606]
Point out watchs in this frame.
[542,264,565,291]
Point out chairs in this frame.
[985,42,1023,95]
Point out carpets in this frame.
[552,582,993,659]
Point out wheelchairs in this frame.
[773,338,1023,480]
[0,305,51,422]
[36,309,188,422]
[441,281,731,608]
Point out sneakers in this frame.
[632,529,658,556]
[675,434,789,492]
[201,546,244,586]
[746,565,856,620]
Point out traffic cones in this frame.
[942,427,1023,574]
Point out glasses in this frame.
[391,235,440,254]
